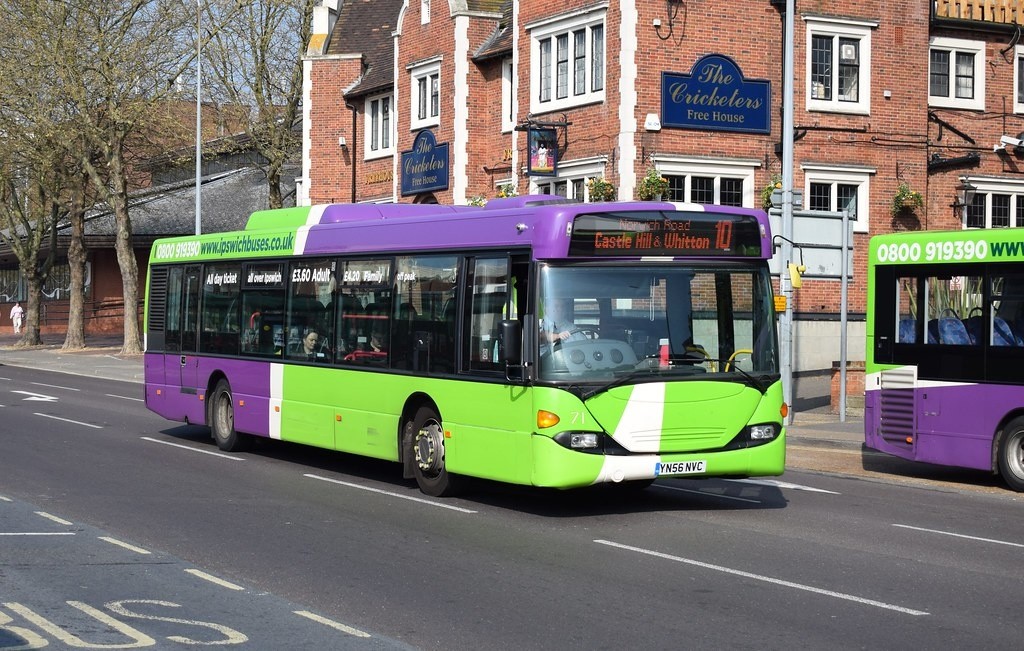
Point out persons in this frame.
[10,303,24,334]
[289,289,585,366]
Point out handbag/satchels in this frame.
[21,312,26,321]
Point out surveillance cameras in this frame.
[1001,136,1023,146]
[339,137,346,148]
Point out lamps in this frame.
[949,175,978,218]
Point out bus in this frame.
[144,195,805,497]
[864,227,1023,496]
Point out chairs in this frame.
[967,307,984,346]
[938,308,972,345]
[364,304,400,363]
[343,301,366,330]
[993,308,1018,346]
[397,303,418,354]
[899,314,938,344]
[438,297,457,363]
[310,301,326,320]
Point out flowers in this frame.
[890,181,925,219]
[466,194,489,207]
[637,162,671,201]
[585,176,616,201]
[495,182,517,198]
[760,173,783,213]
